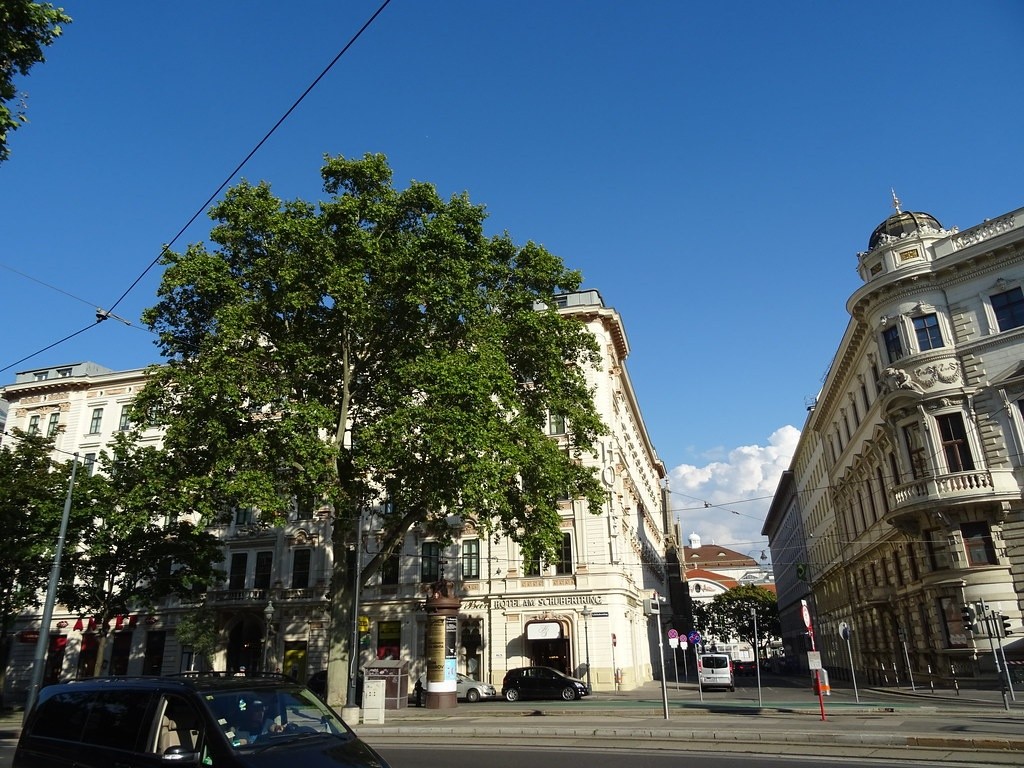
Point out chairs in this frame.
[160,707,204,758]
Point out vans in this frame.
[698,653,735,692]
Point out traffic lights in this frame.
[996,614,1013,637]
[960,601,979,637]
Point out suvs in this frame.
[501,666,590,701]
[413,672,497,703]
[12,668,394,768]
[738,662,757,676]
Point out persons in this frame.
[231,697,292,755]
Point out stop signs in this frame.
[612,634,617,647]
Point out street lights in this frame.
[581,606,592,695]
[262,600,275,677]
